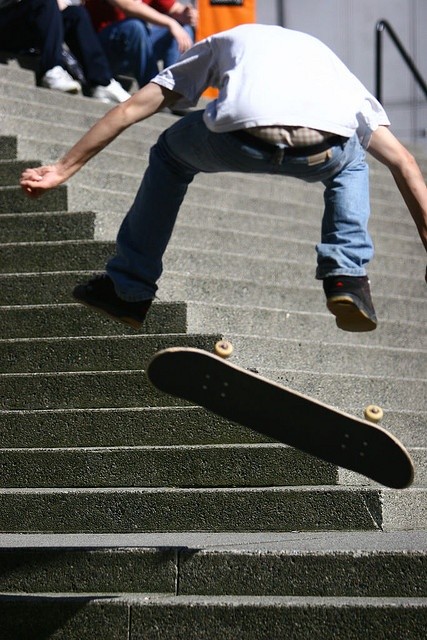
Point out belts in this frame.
[229,130,349,158]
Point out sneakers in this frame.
[73,273,158,329]
[323,275,377,332]
[38,66,80,93]
[91,78,132,102]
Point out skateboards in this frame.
[145,339,415,488]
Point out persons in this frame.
[0,1,134,102]
[18,23,427,333]
[81,1,199,115]
